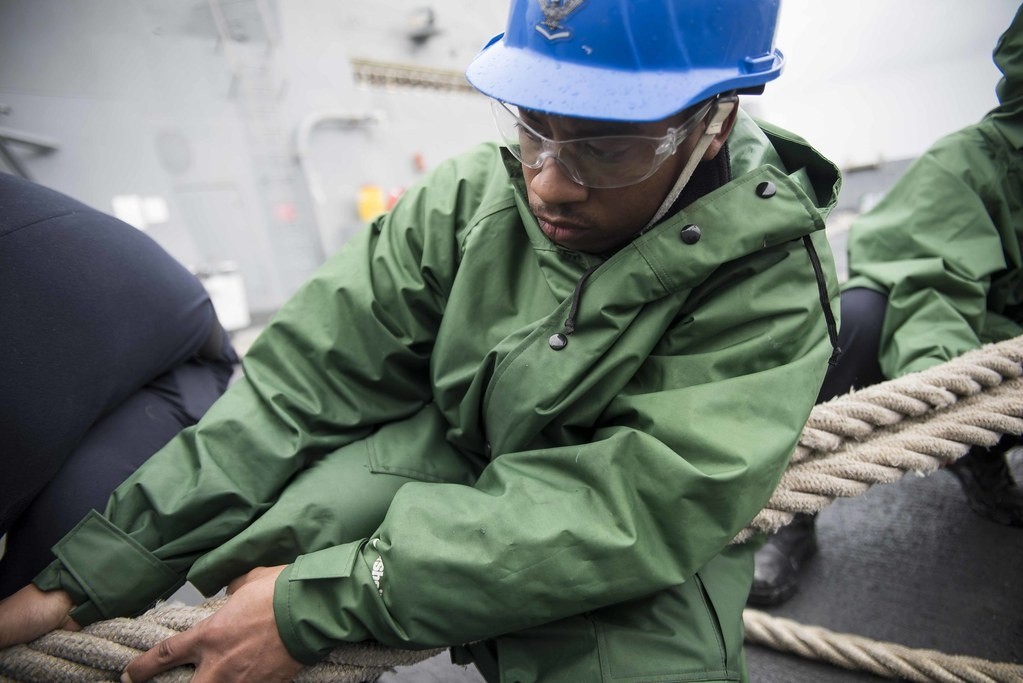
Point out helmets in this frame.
[465,0,785,120]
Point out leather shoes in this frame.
[944,447,1023,526]
[746,509,820,604]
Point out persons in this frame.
[745,0,1023,609]
[0,0,841,683]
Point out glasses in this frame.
[490,95,720,188]
